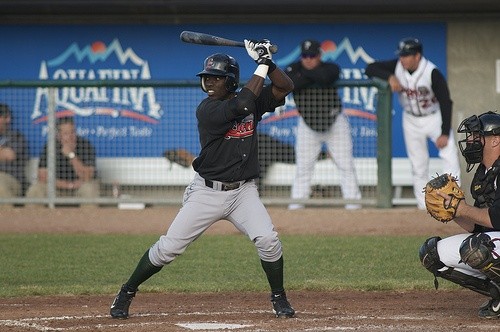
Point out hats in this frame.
[301,40,321,54]
[393,37,422,56]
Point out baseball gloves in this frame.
[422,172,466,224]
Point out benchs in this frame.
[22,157,445,206]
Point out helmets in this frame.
[476,113,500,135]
[195,53,240,85]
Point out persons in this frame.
[284,37,363,210]
[109,39,296,320]
[365,37,462,212]
[420,110,500,319]
[0,102,31,208]
[24,116,102,209]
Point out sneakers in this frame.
[270,292,295,318]
[110,284,136,320]
[477,298,500,319]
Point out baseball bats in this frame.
[179,30,279,54]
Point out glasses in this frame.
[301,54,318,58]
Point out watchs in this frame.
[66,151,76,161]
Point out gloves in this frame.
[244,39,263,65]
[252,41,272,80]
